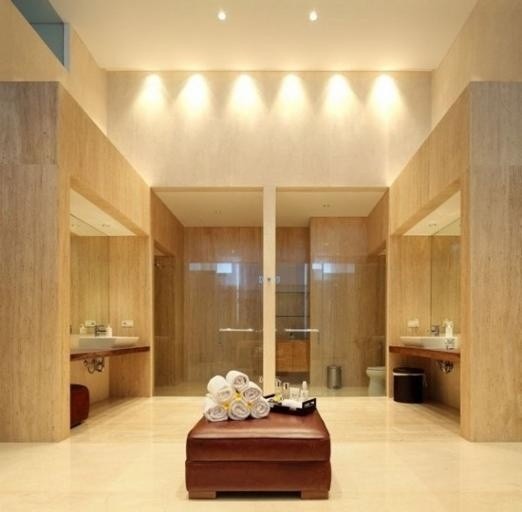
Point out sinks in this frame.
[399,335,458,348]
[77,336,141,350]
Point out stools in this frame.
[70,384,89,428]
[183,409,332,501]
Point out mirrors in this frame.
[69,211,113,336]
[428,216,463,336]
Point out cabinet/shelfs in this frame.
[276,339,310,373]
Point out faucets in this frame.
[93,323,108,337]
[430,323,440,337]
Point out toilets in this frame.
[365,365,387,397]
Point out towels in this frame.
[203,370,272,424]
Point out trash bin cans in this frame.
[392,367,429,403]
[327,364,341,389]
